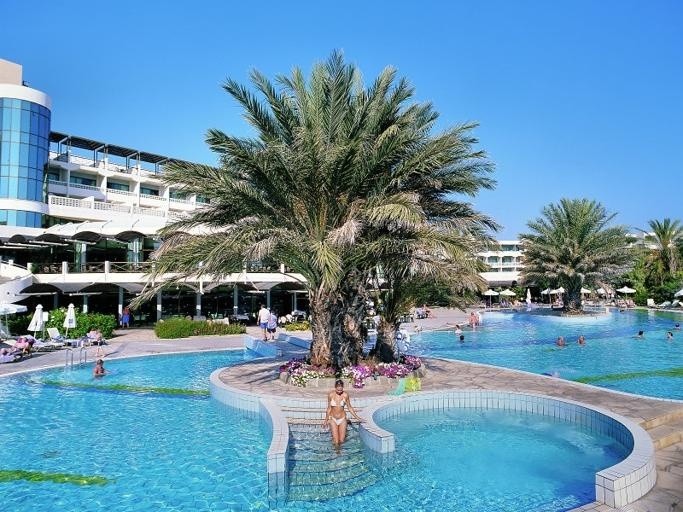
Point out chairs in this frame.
[0,325,106,361]
[617,299,683,309]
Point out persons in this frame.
[121,304,130,329]
[267,310,278,340]
[634,330,644,339]
[256,303,271,341]
[455,311,479,342]
[0,337,29,355]
[321,378,367,454]
[665,331,673,341]
[673,322,681,330]
[556,336,565,346]
[576,335,585,345]
[93,358,110,377]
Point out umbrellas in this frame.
[0,303,28,339]
[481,288,499,307]
[556,286,565,301]
[26,303,44,338]
[499,289,516,301]
[540,287,557,303]
[62,302,77,336]
[580,287,591,296]
[526,287,532,304]
[616,285,637,299]
